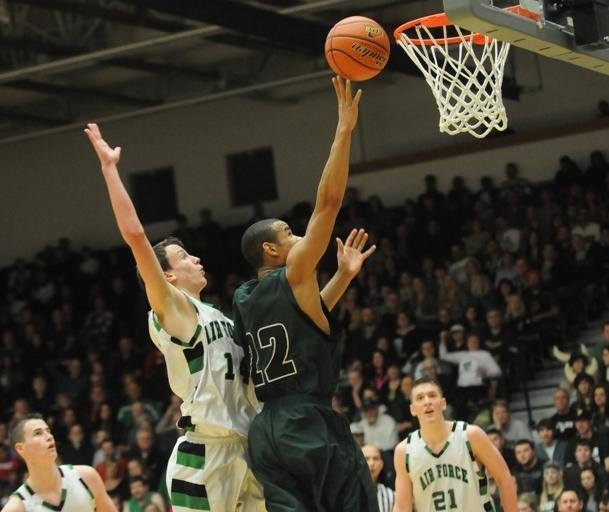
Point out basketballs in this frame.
[325,16,390,81]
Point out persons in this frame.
[0,149,609,512]
[233,74,380,511]
[81,121,378,510]
